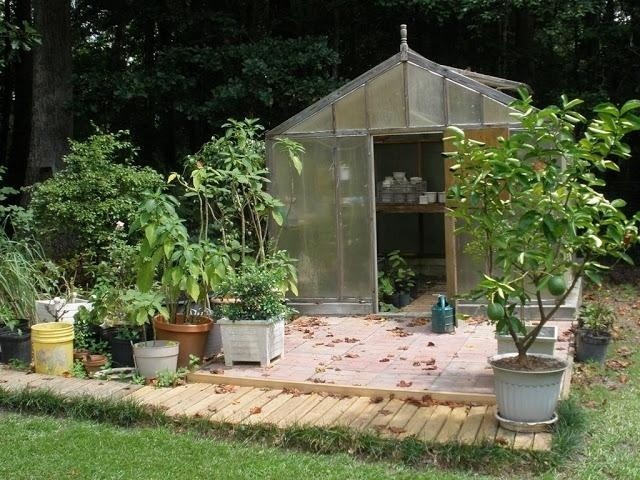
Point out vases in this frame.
[378,250,416,314]
[377,171,445,205]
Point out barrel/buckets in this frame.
[31,322,75,377]
